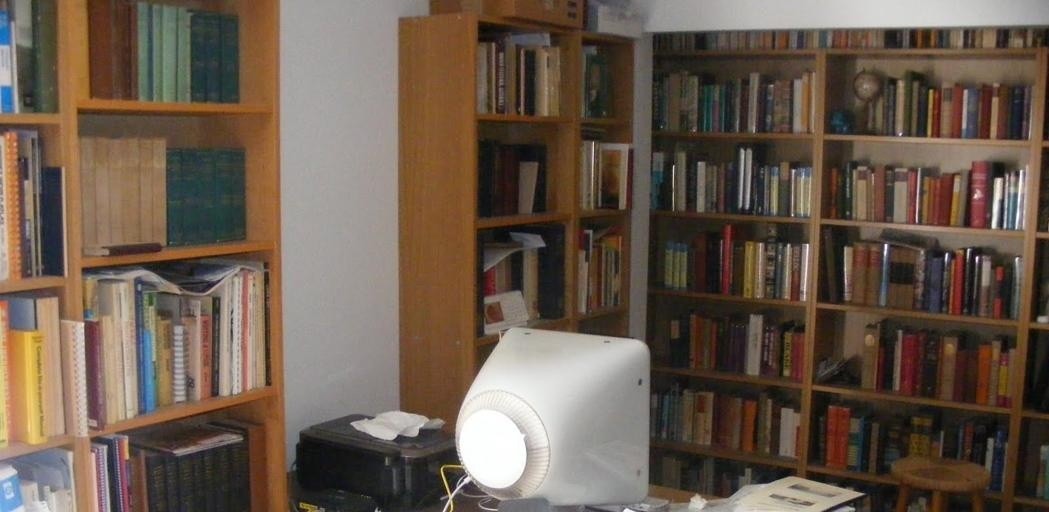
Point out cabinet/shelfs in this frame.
[643,46,819,500]
[399,20,637,433]
[0,0,286,510]
[1006,50,1047,511]
[798,49,1043,512]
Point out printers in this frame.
[290,413,451,511]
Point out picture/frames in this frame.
[598,141,629,209]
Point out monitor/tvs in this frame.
[453,322,652,507]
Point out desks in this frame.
[287,468,729,512]
